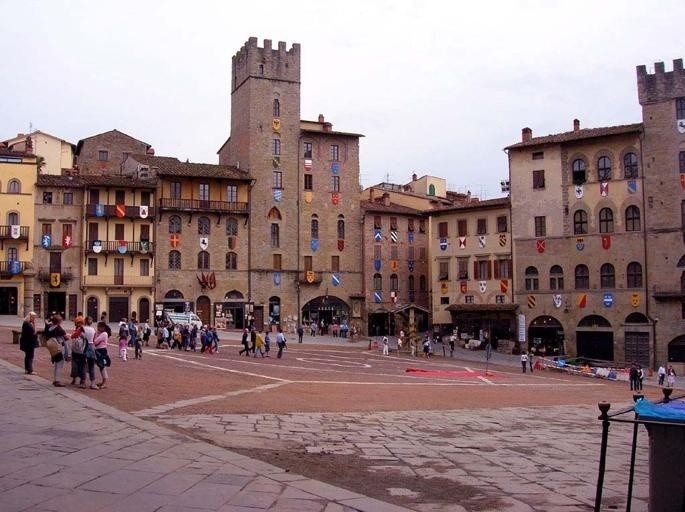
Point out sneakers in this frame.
[52,381,106,389]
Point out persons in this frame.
[484,334,492,360]
[528,351,535,373]
[630,363,677,389]
[521,350,528,373]
[17,310,458,391]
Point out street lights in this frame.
[294,278,301,328]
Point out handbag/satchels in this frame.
[46,337,62,356]
[71,336,87,354]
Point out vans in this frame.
[163,311,203,331]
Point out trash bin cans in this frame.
[638,400,685,512]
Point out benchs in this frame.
[12,329,48,347]
